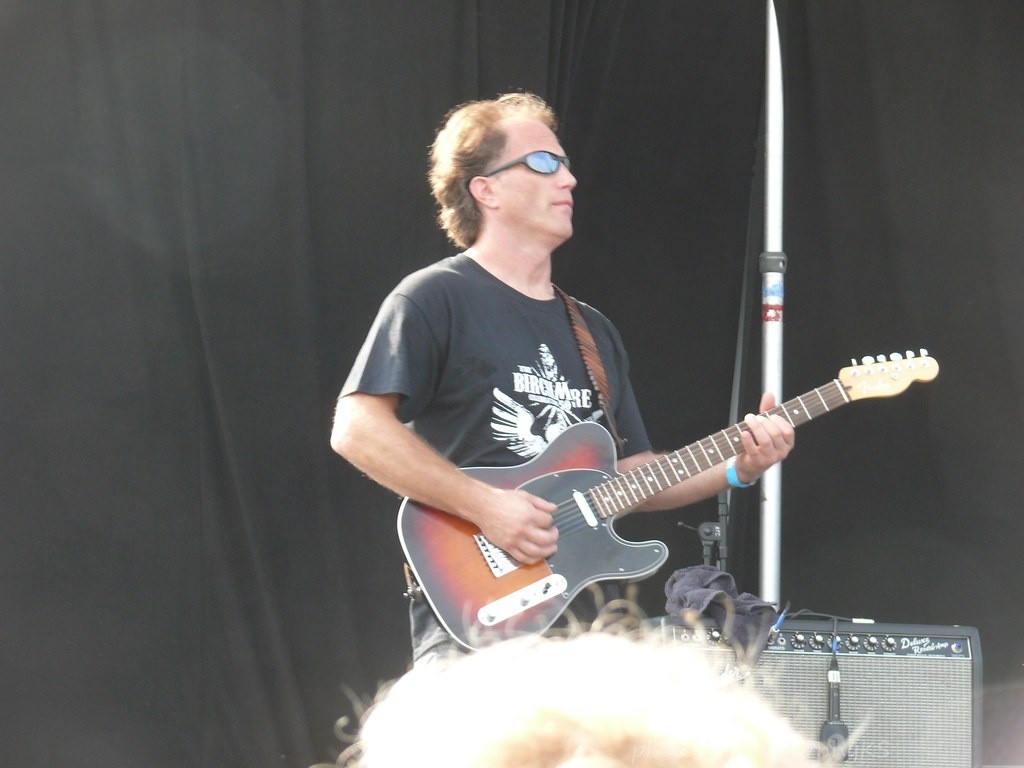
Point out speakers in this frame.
[689,613,981,768]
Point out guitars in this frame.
[392,346,943,659]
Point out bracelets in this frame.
[726,456,756,488]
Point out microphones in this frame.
[816,659,849,762]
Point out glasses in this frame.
[484,150,571,176]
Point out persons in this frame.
[330,96,794,665]
